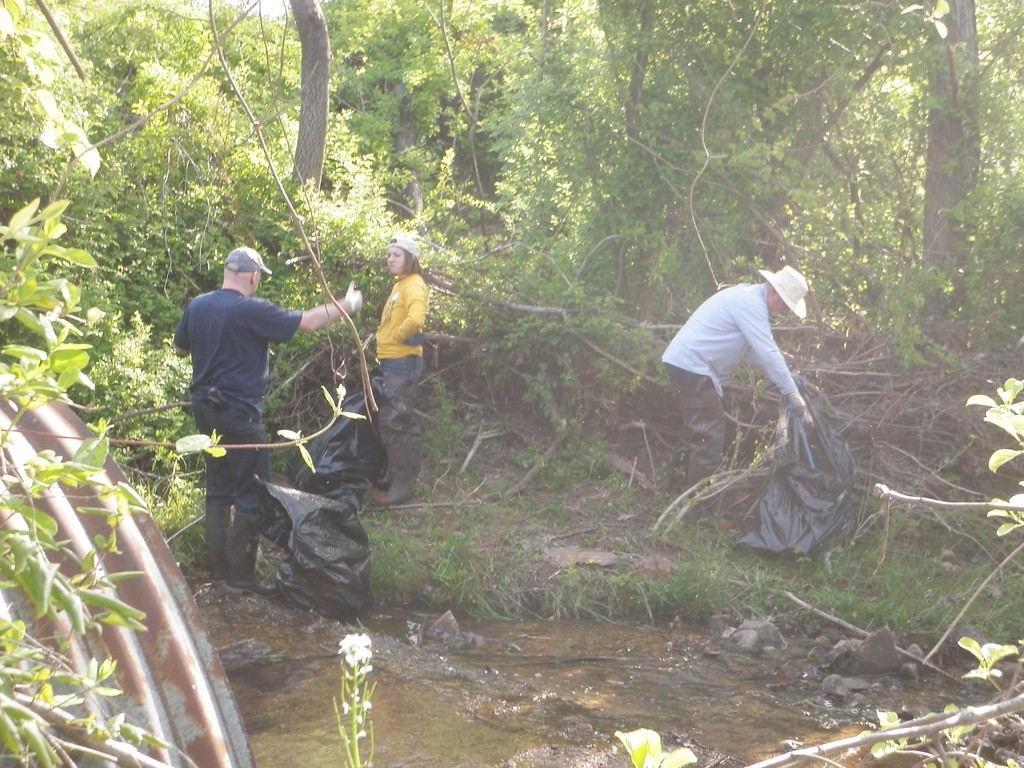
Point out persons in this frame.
[170,246,363,593]
[661,265,810,528]
[369,237,429,504]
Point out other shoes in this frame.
[682,508,736,531]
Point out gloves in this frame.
[786,392,806,417]
[346,281,363,318]
[406,333,424,346]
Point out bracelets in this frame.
[344,296,357,314]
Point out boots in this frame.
[205,505,231,581]
[225,512,281,599]
[373,441,421,506]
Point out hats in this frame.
[387,231,419,258]
[224,246,274,275]
[758,265,810,319]
